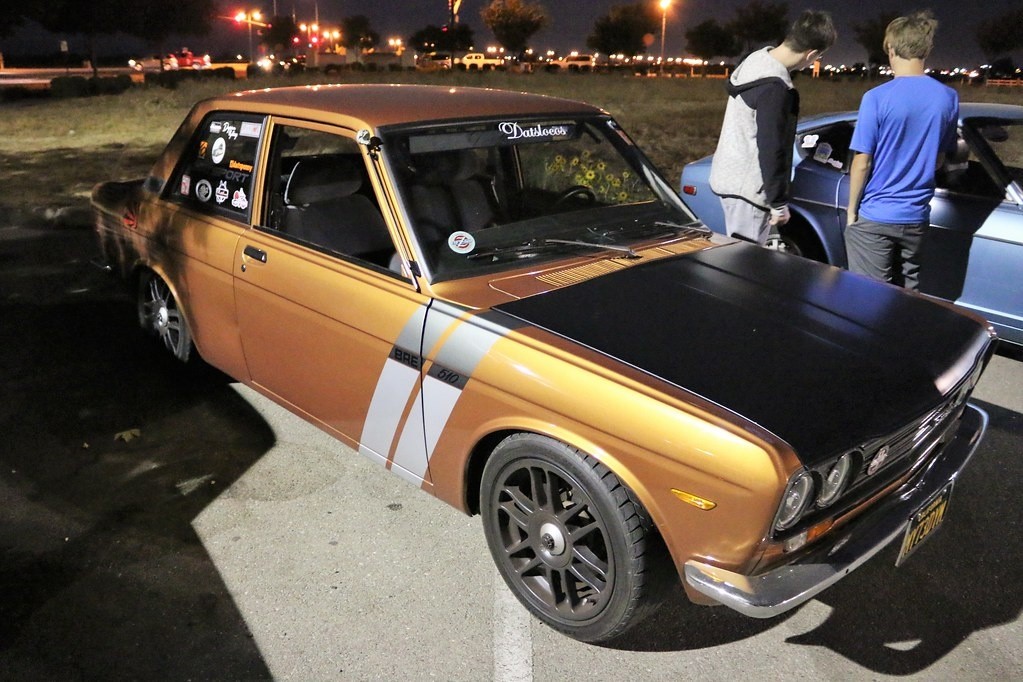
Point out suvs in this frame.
[549,55,593,73]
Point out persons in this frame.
[708,7,837,245]
[845,13,960,291]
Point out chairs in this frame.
[945,139,975,193]
[410,149,494,241]
[281,155,395,255]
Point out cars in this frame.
[89,83,1000,646]
[679,102,1023,347]
[130,52,205,73]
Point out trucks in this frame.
[462,52,505,71]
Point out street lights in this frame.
[659,0,671,75]
[235,12,261,63]
